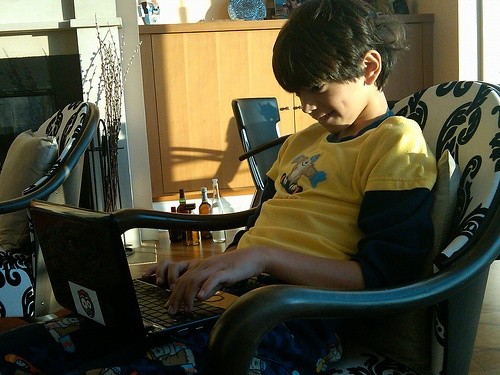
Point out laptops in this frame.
[30,198,242,345]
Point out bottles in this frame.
[176,188,189,240]
[178,0,188,23]
[208,179,226,242]
[186,208,199,246]
[197,187,212,239]
[168,207,182,243]
[273,0,286,19]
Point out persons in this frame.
[0,0,438,375]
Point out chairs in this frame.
[207,81,500,375]
[1,102,99,317]
[230,98,295,248]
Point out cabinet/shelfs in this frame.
[140,12,435,205]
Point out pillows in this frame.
[357,150,461,371]
[1,130,63,254]
